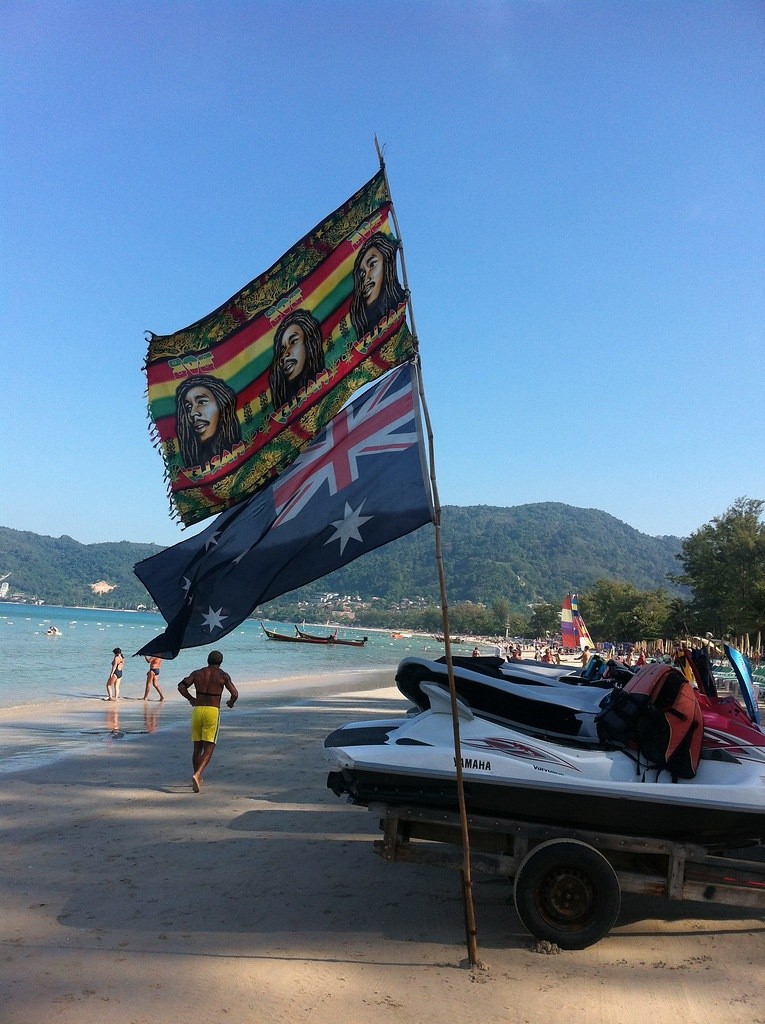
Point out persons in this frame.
[138,655,164,702]
[178,650,239,792]
[102,647,125,700]
[473,642,674,668]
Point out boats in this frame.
[388,629,414,640]
[261,622,338,644]
[321,679,765,859]
[392,639,765,765]
[434,633,466,645]
[293,624,368,647]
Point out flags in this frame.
[560,593,596,650]
[127,360,437,660]
[140,170,419,532]
